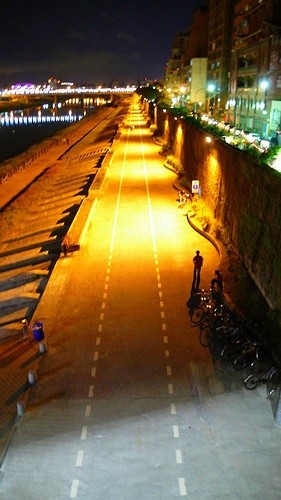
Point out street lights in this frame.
[194,85,215,113]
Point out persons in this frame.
[211,270,223,293]
[193,251,203,282]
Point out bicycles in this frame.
[189,288,281,396]
